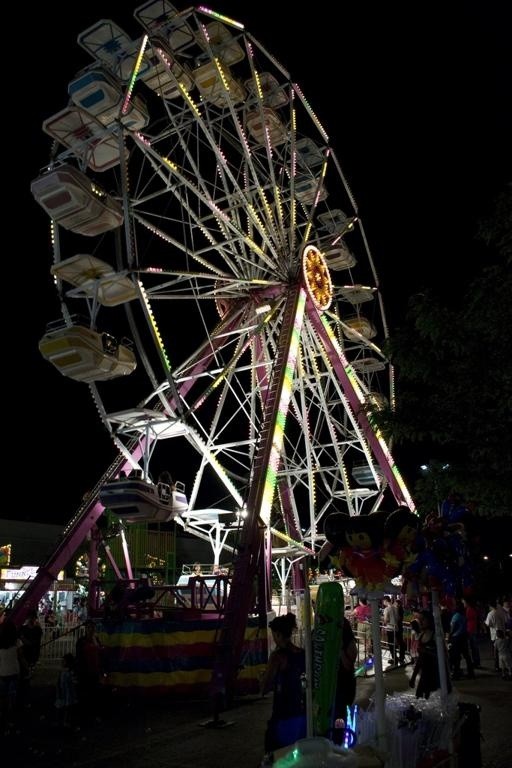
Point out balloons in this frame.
[319,500,483,613]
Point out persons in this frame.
[1,622,27,737]
[381,587,512,699]
[75,618,108,726]
[353,598,373,624]
[191,560,203,576]
[158,469,177,493]
[56,651,81,731]
[258,612,306,767]
[0,594,88,638]
[310,581,360,747]
